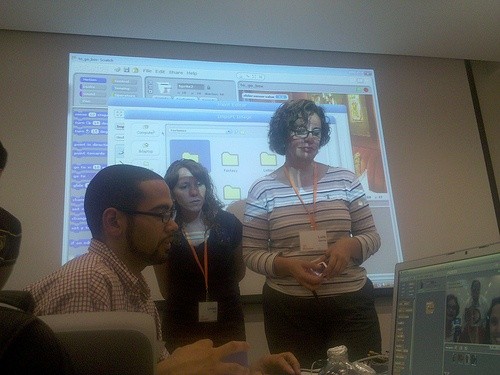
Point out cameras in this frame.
[310,260,329,279]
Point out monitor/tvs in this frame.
[389,244,500,375]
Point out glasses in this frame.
[127,208,177,224]
[290,127,323,138]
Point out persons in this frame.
[462,279,489,343]
[242,98,383,371]
[485,297,500,345]
[153,158,248,369]
[446,294,459,342]
[24,163,302,375]
[0,206,251,375]
